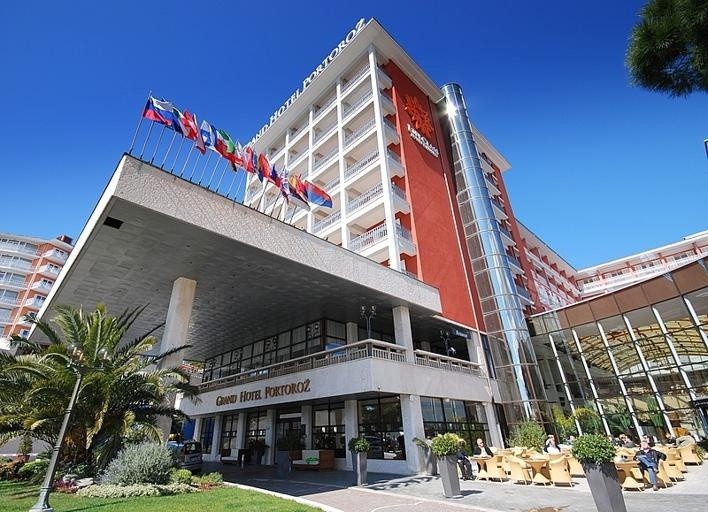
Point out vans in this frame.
[167,438,203,472]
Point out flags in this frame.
[142,96,333,213]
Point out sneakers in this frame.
[655,469,658,474]
[653,485,659,491]
[463,476,472,480]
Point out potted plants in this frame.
[348,438,370,485]
[572,433,628,512]
[430,433,466,499]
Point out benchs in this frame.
[221,450,251,464]
[292,450,334,471]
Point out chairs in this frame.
[457,443,702,492]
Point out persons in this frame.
[455,450,475,479]
[544,433,676,456]
[472,437,495,479]
[632,440,668,491]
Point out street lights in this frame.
[29,344,114,511]
